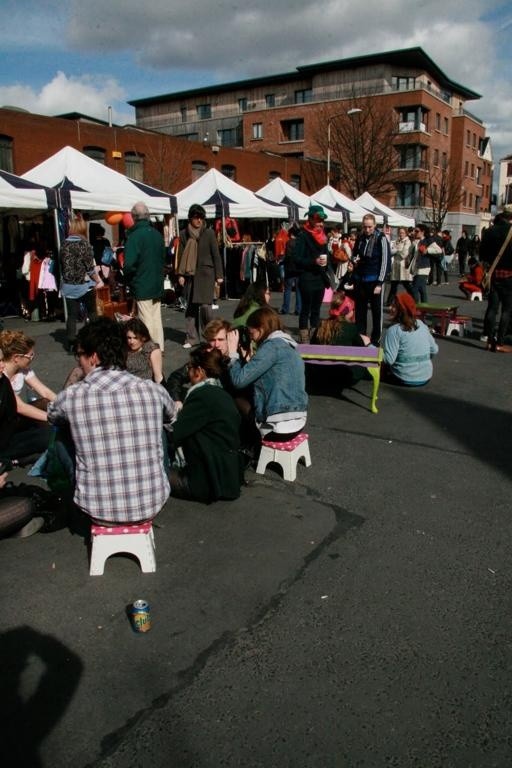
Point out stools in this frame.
[88,523,156,576]
[446,315,472,340]
[255,434,313,484]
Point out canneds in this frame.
[132,599,152,633]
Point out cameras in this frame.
[228,326,250,350]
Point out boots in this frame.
[300,329,310,345]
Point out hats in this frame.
[188,204,206,219]
[397,292,417,318]
[304,206,328,219]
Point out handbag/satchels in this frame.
[0,481,70,532]
[323,269,340,291]
[334,249,348,262]
[481,269,493,297]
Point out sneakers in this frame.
[480,335,497,342]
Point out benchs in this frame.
[291,343,384,416]
[415,302,461,338]
[460,281,482,303]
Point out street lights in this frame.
[325,108,365,183]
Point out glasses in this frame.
[74,351,87,361]
[16,352,35,360]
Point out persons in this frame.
[0,347,20,461]
[1,327,58,463]
[43,316,178,529]
[1,201,512,389]
[167,316,236,411]
[224,306,310,467]
[164,346,246,505]
[0,461,46,542]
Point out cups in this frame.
[319,254,327,266]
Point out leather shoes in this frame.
[487,341,495,351]
[494,344,512,352]
